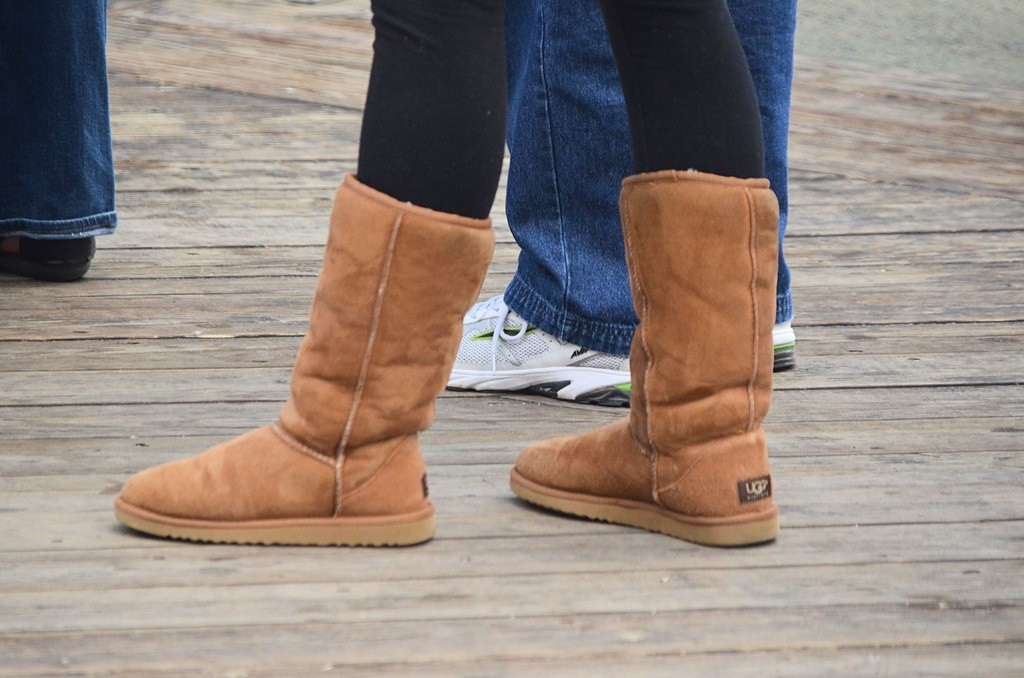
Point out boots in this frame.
[114,174,495,548]
[509,171,779,546]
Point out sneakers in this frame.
[446,295,632,407]
[770,315,796,374]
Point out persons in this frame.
[0,0,117,280]
[114,0,796,546]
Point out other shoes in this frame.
[0,233,96,286]
